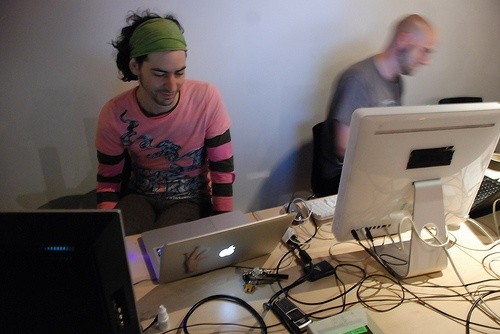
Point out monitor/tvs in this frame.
[0,210,144,334]
[331,101,500,279]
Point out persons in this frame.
[326,14,438,186]
[95,9,236,238]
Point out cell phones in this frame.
[273,298,313,332]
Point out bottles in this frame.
[157,305,171,332]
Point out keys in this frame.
[242,266,289,294]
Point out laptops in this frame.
[140,210,297,283]
[469,177,500,218]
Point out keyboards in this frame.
[304,194,337,221]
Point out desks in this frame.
[122,192,500,334]
